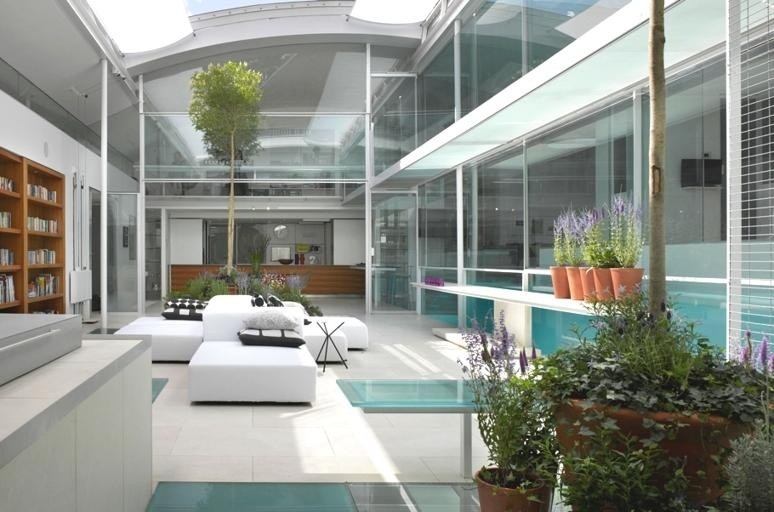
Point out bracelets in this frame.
[278,259,293,264]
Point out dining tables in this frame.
[187,58,255,294]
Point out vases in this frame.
[547,194,642,269]
[490,285,774,512]
[455,303,548,483]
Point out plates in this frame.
[0,146,25,314]
[25,155,66,313]
[411,275,658,362]
[0,333,155,512]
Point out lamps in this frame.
[391,264,416,306]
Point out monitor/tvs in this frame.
[0,174,63,316]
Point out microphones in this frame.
[305,317,369,349]
[298,322,348,363]
[112,316,202,362]
[188,340,317,403]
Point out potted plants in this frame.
[123,226,129,247]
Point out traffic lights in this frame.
[165,298,204,308]
[265,294,284,307]
[237,327,307,347]
[161,308,203,320]
[250,292,266,306]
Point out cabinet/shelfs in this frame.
[294,253,299,265]
[425,275,444,287]
[299,252,305,264]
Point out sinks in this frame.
[201,295,312,341]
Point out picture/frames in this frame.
[145,377,495,512]
[350,266,398,308]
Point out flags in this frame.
[552,394,774,512]
[474,467,554,512]
[549,267,645,301]
